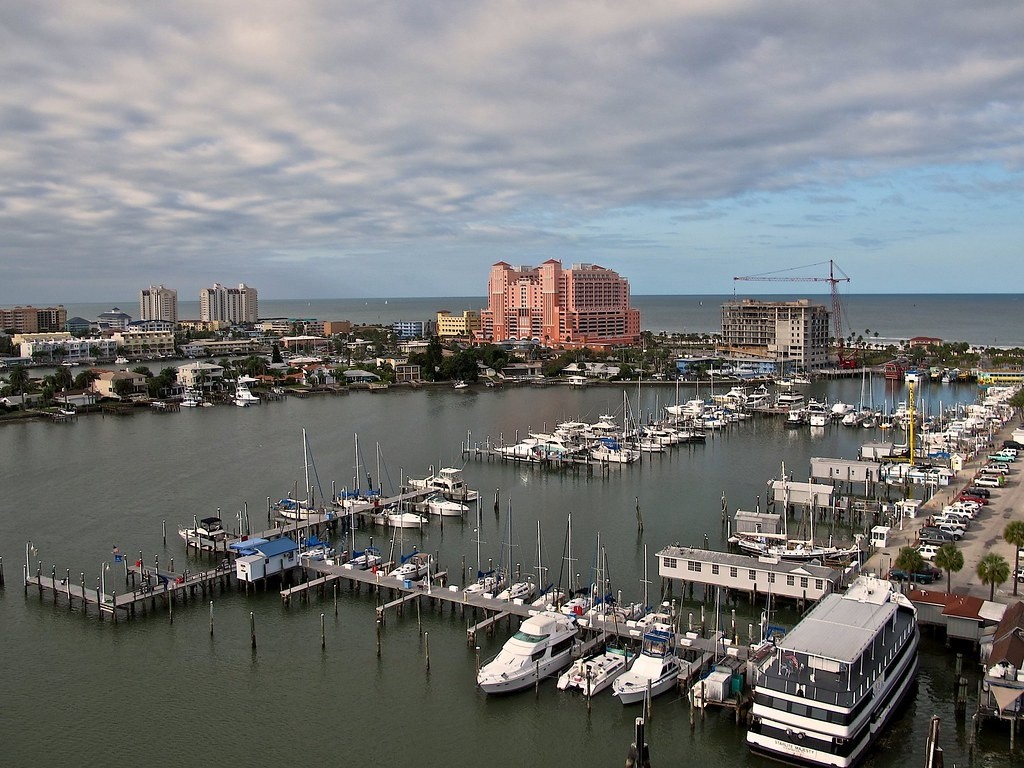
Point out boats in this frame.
[406,466,479,501]
[407,492,469,518]
[177,506,241,554]
[300,545,336,567]
[343,553,381,571]
[52,349,267,424]
[455,364,955,768]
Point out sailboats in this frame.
[386,469,434,581]
[337,432,375,513]
[371,441,427,526]
[277,426,330,519]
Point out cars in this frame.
[884,439,1024,589]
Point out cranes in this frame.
[729,258,852,345]
